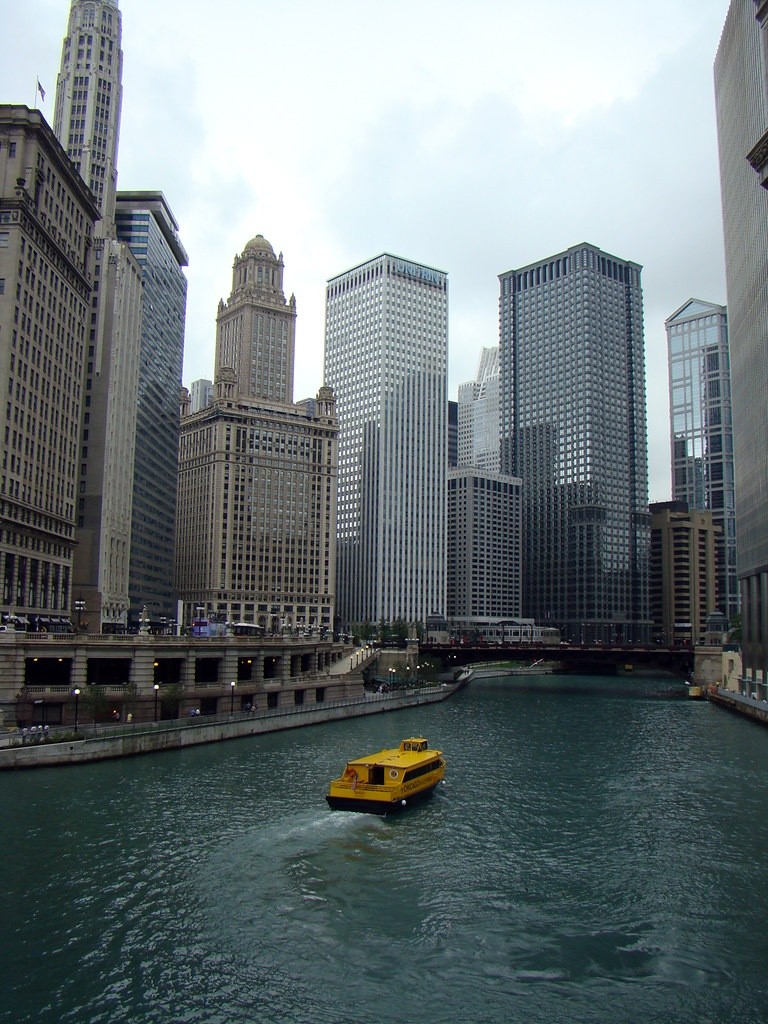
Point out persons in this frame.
[191,708,200,717]
[241,702,258,711]
[127,712,132,723]
[113,712,120,723]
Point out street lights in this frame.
[196,606,205,635]
[75,592,86,633]
[389,668,392,693]
[73,689,81,734]
[154,684,160,722]
[407,667,410,689]
[230,682,236,714]
[392,668,396,692]
[417,665,419,688]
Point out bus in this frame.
[232,623,265,638]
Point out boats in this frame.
[324,736,447,815]
